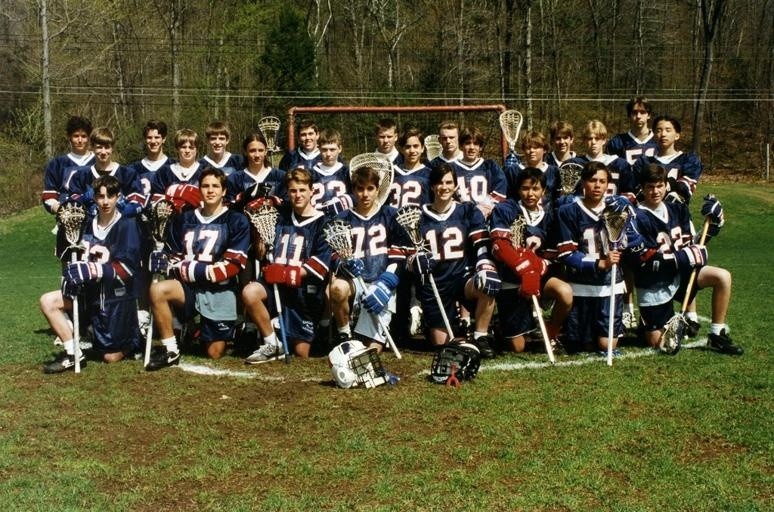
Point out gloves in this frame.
[61,276,81,300]
[408,252,436,274]
[167,184,203,207]
[349,260,366,277]
[518,268,541,299]
[474,264,502,297]
[242,182,272,203]
[148,250,168,275]
[63,261,103,286]
[504,150,520,167]
[361,272,400,314]
[175,258,210,284]
[701,193,726,227]
[263,263,301,287]
[683,243,708,267]
[607,196,637,219]
[605,192,636,214]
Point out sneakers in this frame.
[335,331,351,346]
[471,334,496,358]
[683,316,701,337]
[144,350,182,371]
[245,340,286,365]
[707,328,744,355]
[43,353,90,373]
[544,331,568,356]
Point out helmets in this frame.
[328,338,389,388]
[431,337,482,385]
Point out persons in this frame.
[384,128,433,343]
[145,169,250,371]
[556,161,643,354]
[243,170,330,364]
[544,120,584,195]
[442,128,506,220]
[129,118,175,200]
[151,127,209,210]
[42,116,98,287]
[40,177,148,373]
[505,130,562,220]
[306,129,355,218]
[405,164,499,360]
[318,167,405,355]
[431,121,466,172]
[70,126,143,218]
[487,169,576,358]
[278,122,344,173]
[624,157,745,355]
[195,122,242,180]
[373,118,402,170]
[227,133,291,261]
[629,117,700,341]
[601,99,660,189]
[576,118,634,220]
